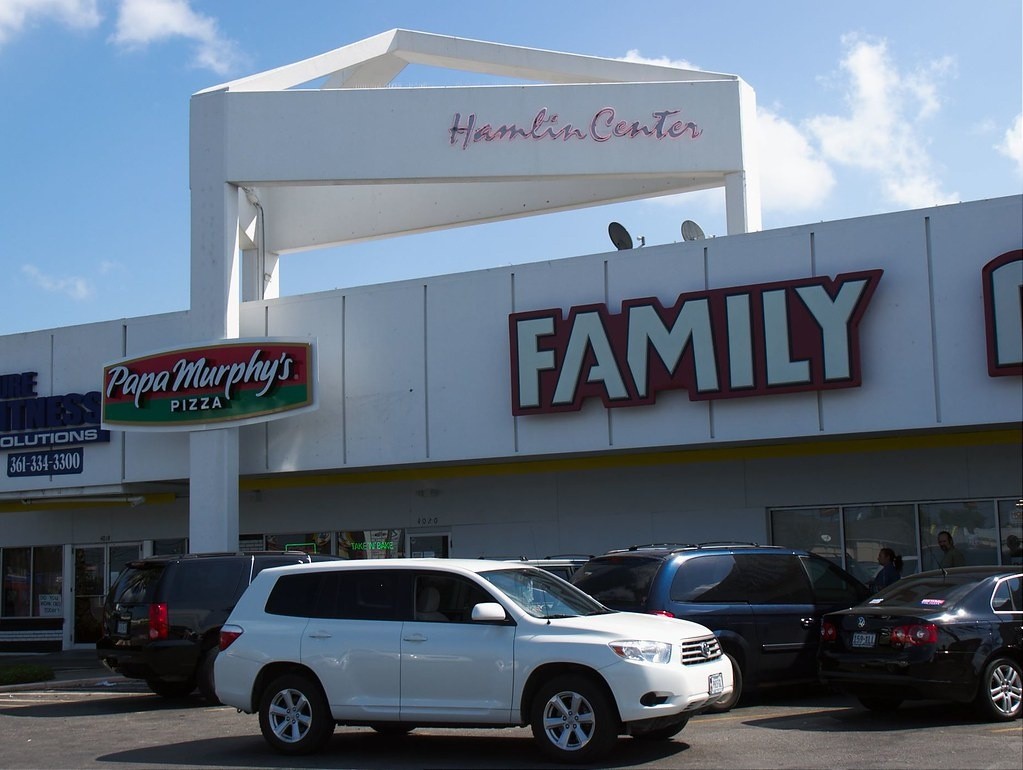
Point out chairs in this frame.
[418,588,451,622]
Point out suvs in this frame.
[212,557,735,759]
[93,553,347,705]
[551,542,877,710]
[5,579,44,617]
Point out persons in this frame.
[869,548,904,593]
[1004,535,1023,565]
[937,531,969,568]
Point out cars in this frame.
[815,544,1023,592]
[806,563,1023,721]
[492,556,591,599]
